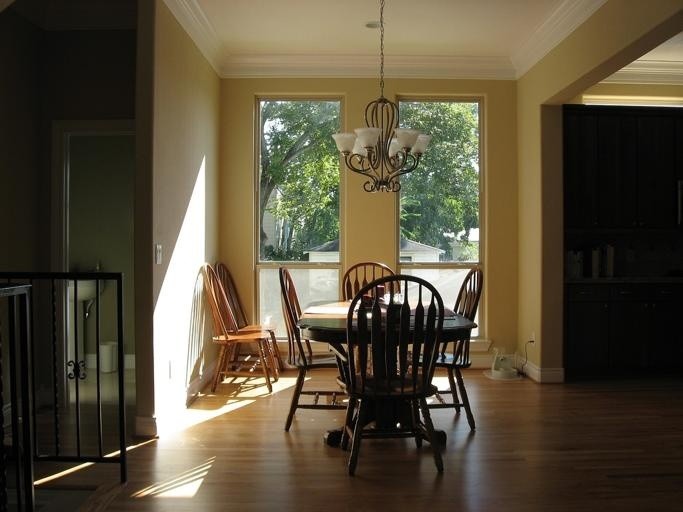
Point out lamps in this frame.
[332,0,432,192]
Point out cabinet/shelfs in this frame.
[568,283,610,372]
[598,109,678,228]
[563,106,598,230]
[610,284,683,373]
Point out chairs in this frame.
[341,273,445,477]
[341,261,400,300]
[203,263,278,394]
[216,261,284,382]
[418,268,483,432]
[278,266,359,432]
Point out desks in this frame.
[296,298,476,450]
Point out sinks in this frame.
[67,279,104,301]
